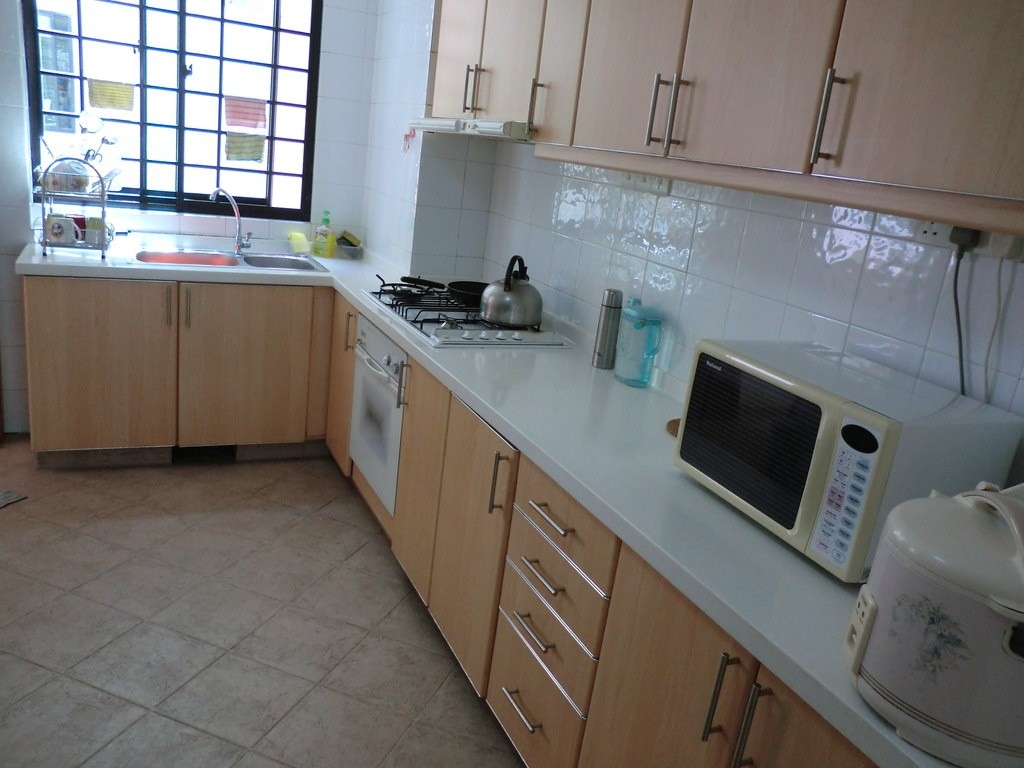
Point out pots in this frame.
[400,276,490,307]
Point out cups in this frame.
[47,212,116,247]
[41,162,89,192]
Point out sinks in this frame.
[243,256,315,270]
[137,251,238,266]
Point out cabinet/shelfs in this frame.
[576,545,877,768]
[486,454,621,768]
[324,289,359,476]
[573,1,844,204]
[527,0,591,167]
[426,393,520,698]
[391,354,452,608]
[31,154,112,260]
[809,0,1024,237]
[433,0,546,124]
[24,275,314,454]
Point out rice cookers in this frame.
[846,479,1023,767]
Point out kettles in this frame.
[479,255,543,327]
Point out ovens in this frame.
[348,314,408,518]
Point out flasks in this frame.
[592,288,623,369]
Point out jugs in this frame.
[612,297,664,388]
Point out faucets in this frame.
[208,187,252,255]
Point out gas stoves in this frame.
[360,281,567,349]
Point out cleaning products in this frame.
[311,209,337,258]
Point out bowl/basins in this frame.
[335,244,363,261]
[287,240,314,257]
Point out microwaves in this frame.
[672,338,1023,587]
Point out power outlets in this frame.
[917,221,1021,260]
[620,169,673,196]
